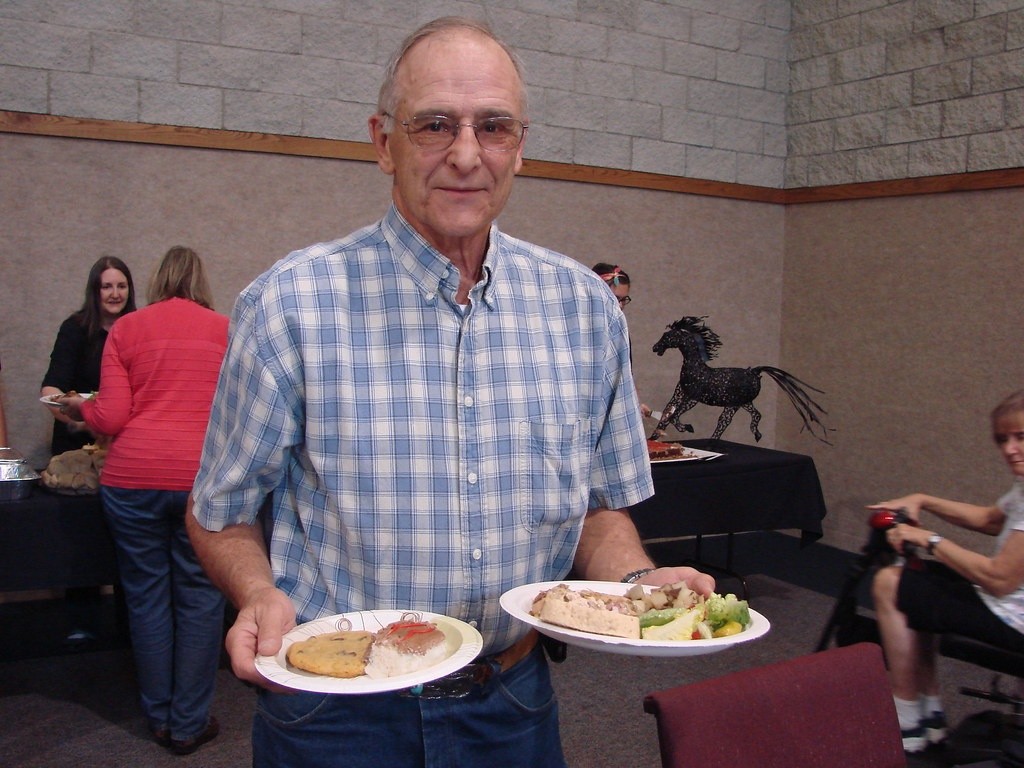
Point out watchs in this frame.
[926,535,941,556]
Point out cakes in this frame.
[646,438,685,460]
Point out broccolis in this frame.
[640,594,749,641]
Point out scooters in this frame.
[813,505,1024,768]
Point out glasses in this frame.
[379,111,529,152]
[616,295,631,305]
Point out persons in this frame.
[59,246,227,756]
[864,384,1024,768]
[39,252,139,459]
[590,264,653,420]
[184,22,716,768]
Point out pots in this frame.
[0,447,41,500]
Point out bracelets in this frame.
[622,565,656,585]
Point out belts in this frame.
[393,628,541,700]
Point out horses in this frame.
[647,314,837,451]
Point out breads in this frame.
[41,449,107,491]
[525,588,640,639]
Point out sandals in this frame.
[901,720,932,757]
[920,711,949,748]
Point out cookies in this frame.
[287,631,377,678]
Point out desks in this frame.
[0,468,136,593]
[625,438,828,579]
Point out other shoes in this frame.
[154,728,171,746]
[170,717,220,756]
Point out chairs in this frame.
[938,631,1024,768]
[646,642,910,768]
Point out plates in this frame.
[500,579,770,656]
[40,393,98,407]
[255,608,483,694]
